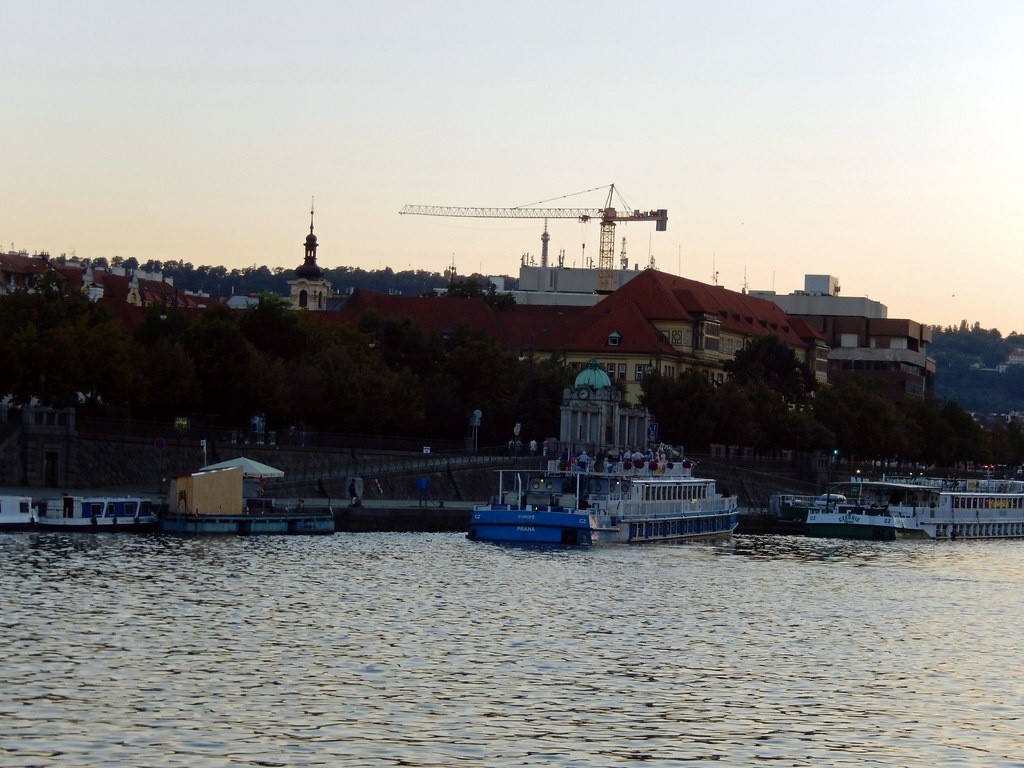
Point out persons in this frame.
[350,477,360,498]
[418,474,426,505]
[951,473,1012,494]
[258,478,269,496]
[431,471,442,502]
[506,435,657,473]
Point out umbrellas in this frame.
[201,455,286,478]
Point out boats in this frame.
[147,455,340,542]
[26,493,151,528]
[464,441,740,556]
[764,465,1024,546]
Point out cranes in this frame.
[393,181,667,299]
